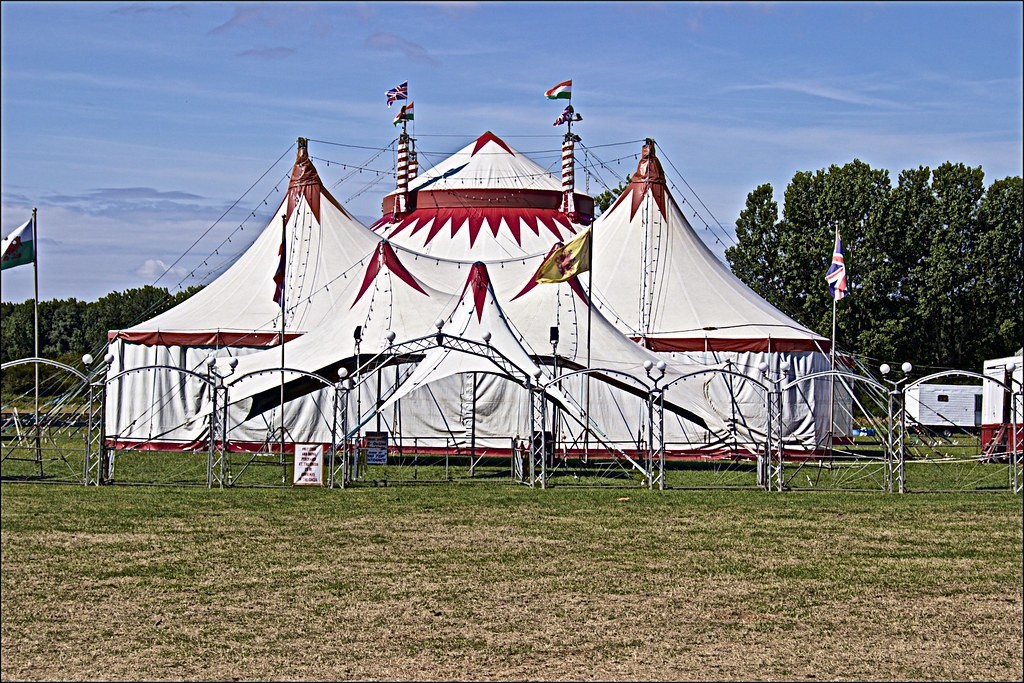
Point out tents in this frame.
[103,130,854,462]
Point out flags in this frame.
[553,106,572,128]
[824,228,850,303]
[0,215,35,272]
[393,102,414,127]
[534,227,590,286]
[544,79,572,101]
[384,80,407,109]
